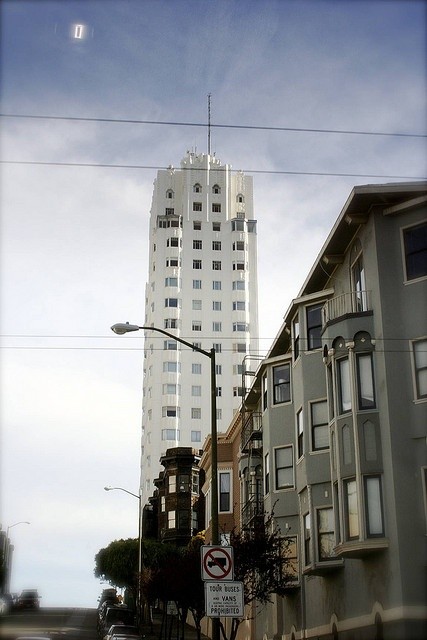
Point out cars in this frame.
[16,589,41,610]
[97,589,147,639]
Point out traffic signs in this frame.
[205,581,243,618]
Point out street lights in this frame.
[104,485,142,617]
[4,521,30,592]
